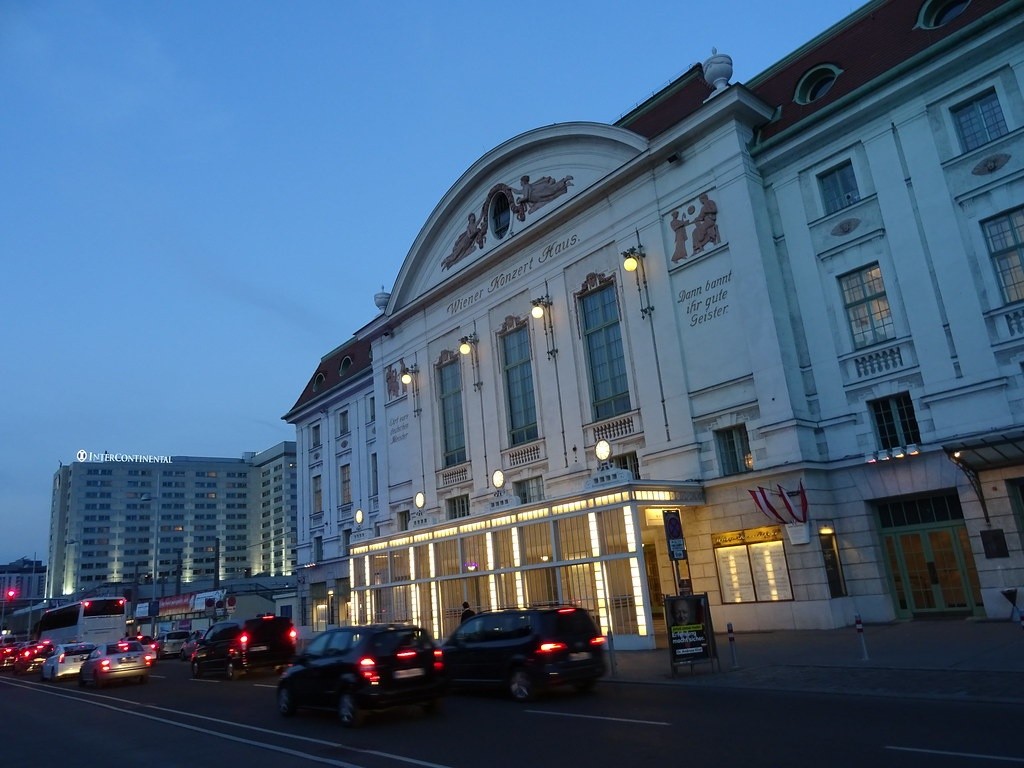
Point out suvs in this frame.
[270,623,447,728]
[153,629,192,660]
[434,602,608,703]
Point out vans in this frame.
[190,616,299,682]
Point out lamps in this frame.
[621,246,637,272]
[457,334,470,355]
[878,449,889,460]
[907,444,919,454]
[401,368,414,384]
[530,296,545,318]
[865,452,876,463]
[892,447,905,457]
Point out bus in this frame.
[33,596,128,646]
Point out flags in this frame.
[747,481,808,523]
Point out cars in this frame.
[120,635,158,661]
[40,642,98,684]
[0,635,56,677]
[79,640,152,690]
[180,629,211,662]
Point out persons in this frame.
[460,602,476,635]
[398,359,407,395]
[507,175,574,202]
[386,367,400,398]
[665,598,709,663]
[690,193,717,256]
[669,211,688,264]
[440,207,483,273]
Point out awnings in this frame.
[941,430,1024,524]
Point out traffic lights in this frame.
[8,590,14,597]
[8,590,15,598]
